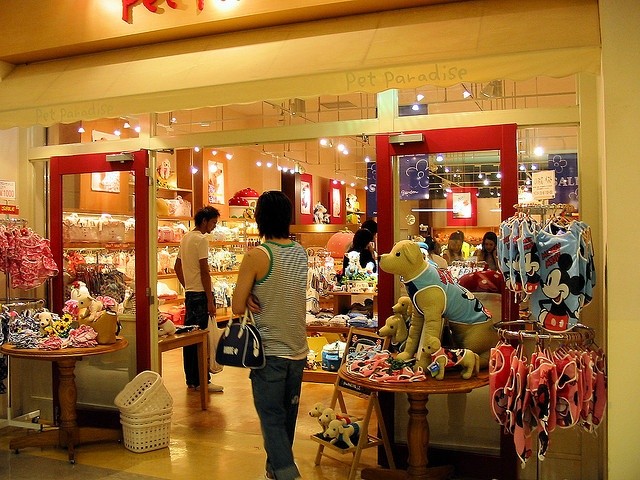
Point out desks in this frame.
[0,335,129,464]
[158,328,210,410]
[338,351,490,480]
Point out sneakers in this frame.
[198,382,221,393]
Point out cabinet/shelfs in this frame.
[63,211,265,322]
[301,287,378,384]
[489,200,606,479]
[1,199,44,435]
[155,146,194,221]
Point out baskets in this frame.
[112,369,175,453]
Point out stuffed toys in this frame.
[77,293,119,321]
[421,337,480,381]
[391,297,412,328]
[34,312,54,328]
[378,315,408,352]
[345,251,368,276]
[378,240,502,372]
[322,420,362,448]
[318,407,358,436]
[309,401,345,433]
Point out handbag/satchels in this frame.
[216,305,266,370]
[78,308,117,343]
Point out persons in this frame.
[343,228,377,274]
[173,206,225,392]
[473,231,500,270]
[232,190,311,477]
[361,220,378,235]
[443,229,465,262]
[424,236,449,270]
